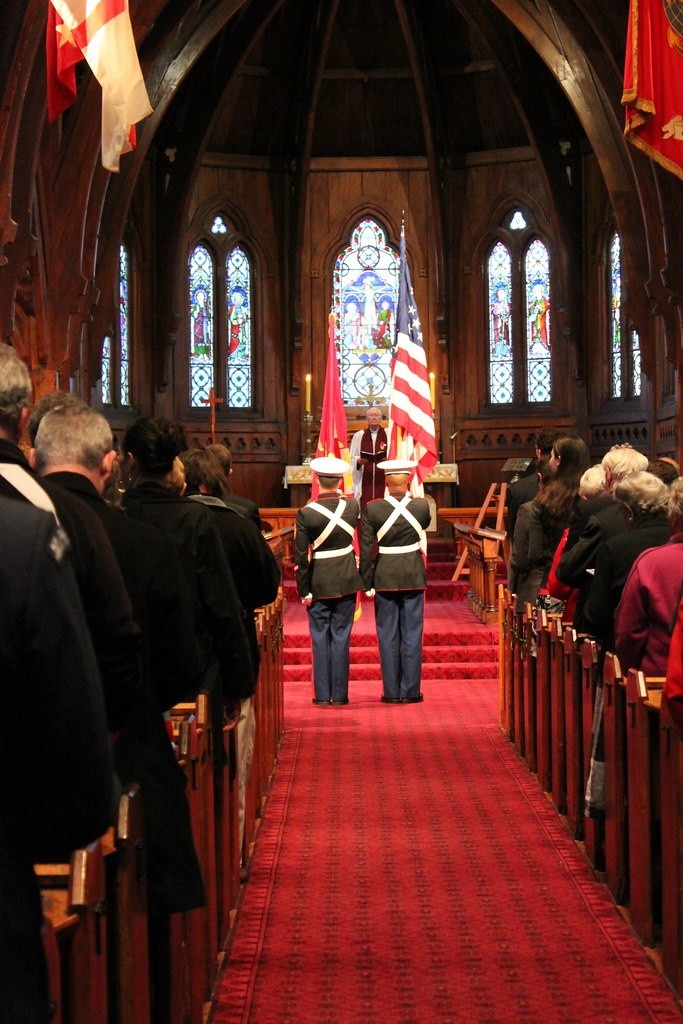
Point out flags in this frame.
[45,0,154,175]
[392,239,439,487]
[308,311,348,504]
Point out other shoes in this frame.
[381,696,400,703]
[332,698,349,705]
[401,693,423,703]
[312,698,330,704]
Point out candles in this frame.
[429,372,436,410]
[305,374,311,413]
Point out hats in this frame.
[376,459,418,474]
[309,457,353,477]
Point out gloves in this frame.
[366,588,376,597]
[300,592,312,606]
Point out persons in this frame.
[293,454,357,704]
[0,339,284,1023]
[350,407,388,498]
[502,425,683,910]
[361,458,431,703]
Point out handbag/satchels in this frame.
[528,595,566,657]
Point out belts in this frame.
[378,541,420,554]
[312,544,354,560]
